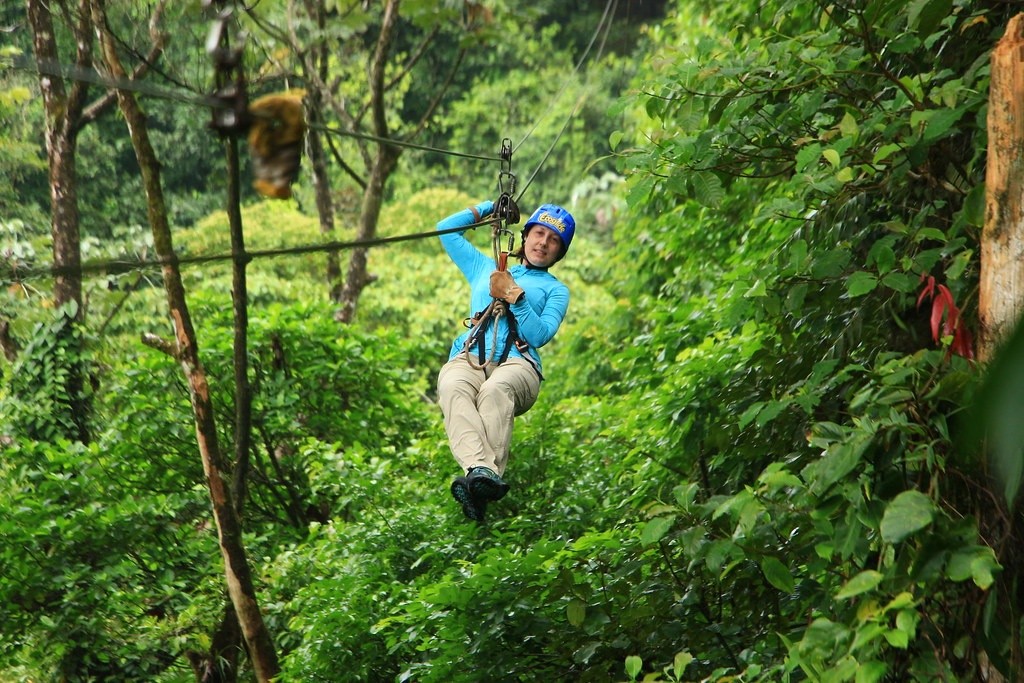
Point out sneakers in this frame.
[450,477,486,521]
[467,473,510,501]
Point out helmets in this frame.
[524,203,576,251]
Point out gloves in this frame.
[488,270,525,304]
[495,195,520,224]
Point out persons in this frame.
[435,195,578,526]
